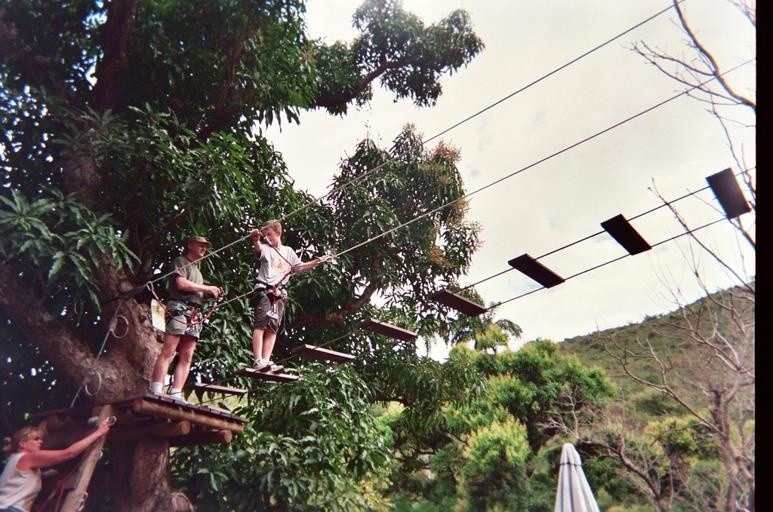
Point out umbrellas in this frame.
[554,443,600,512]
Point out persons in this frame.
[248,219,332,374]
[147,234,225,403]
[0,417,117,512]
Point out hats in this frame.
[186,236,212,249]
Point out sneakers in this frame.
[252,358,284,374]
